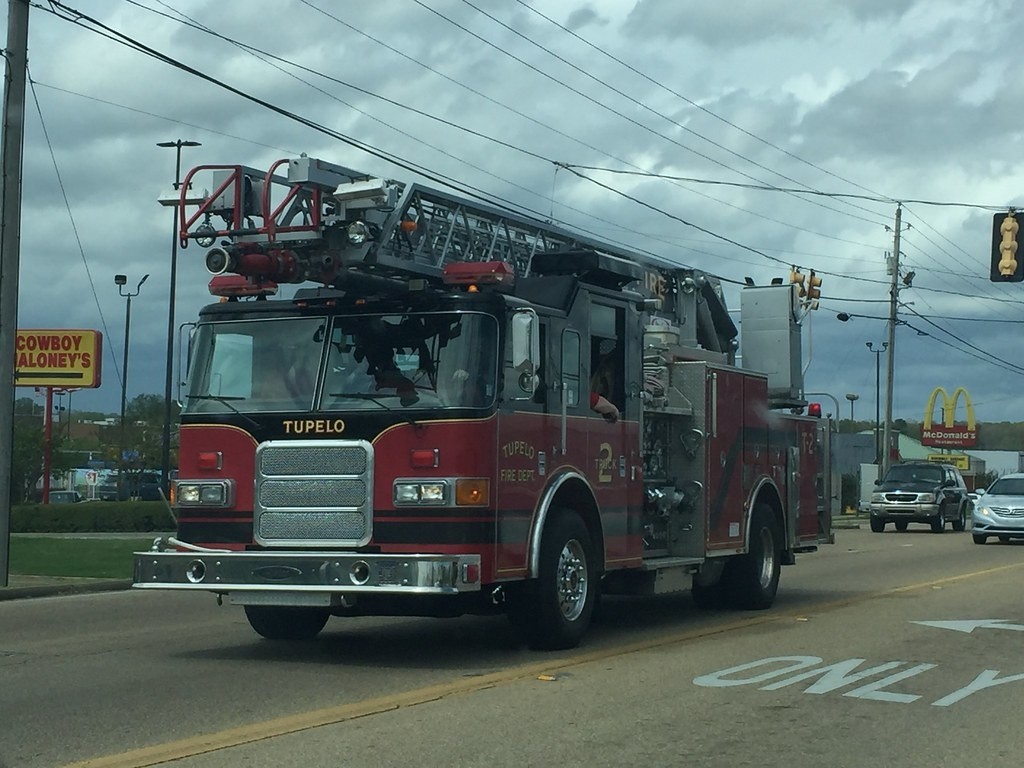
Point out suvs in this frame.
[868,459,969,533]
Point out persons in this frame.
[418,344,479,412]
[270,345,319,402]
[591,392,619,423]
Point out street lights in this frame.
[866,342,888,463]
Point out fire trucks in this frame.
[131,152,837,652]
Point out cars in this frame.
[971,473,1024,544]
